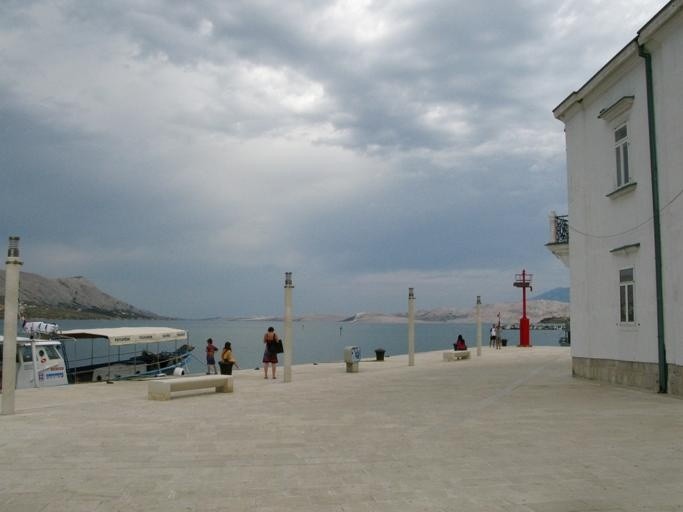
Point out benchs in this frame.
[147,374,235,399]
[442,349,472,362]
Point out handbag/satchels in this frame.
[270,333,277,352]
[277,339,283,353]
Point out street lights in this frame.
[404,286,418,367]
[1,235,27,417]
[280,270,296,382]
[474,294,483,354]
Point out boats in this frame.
[0,335,68,391]
[494,320,561,329]
[23,315,197,385]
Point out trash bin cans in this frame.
[375,348,386,360]
[502,339,507,347]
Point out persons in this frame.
[218,342,241,375]
[207,339,218,374]
[495,327,502,349]
[489,324,496,348]
[262,326,279,378]
[456,335,465,359]
